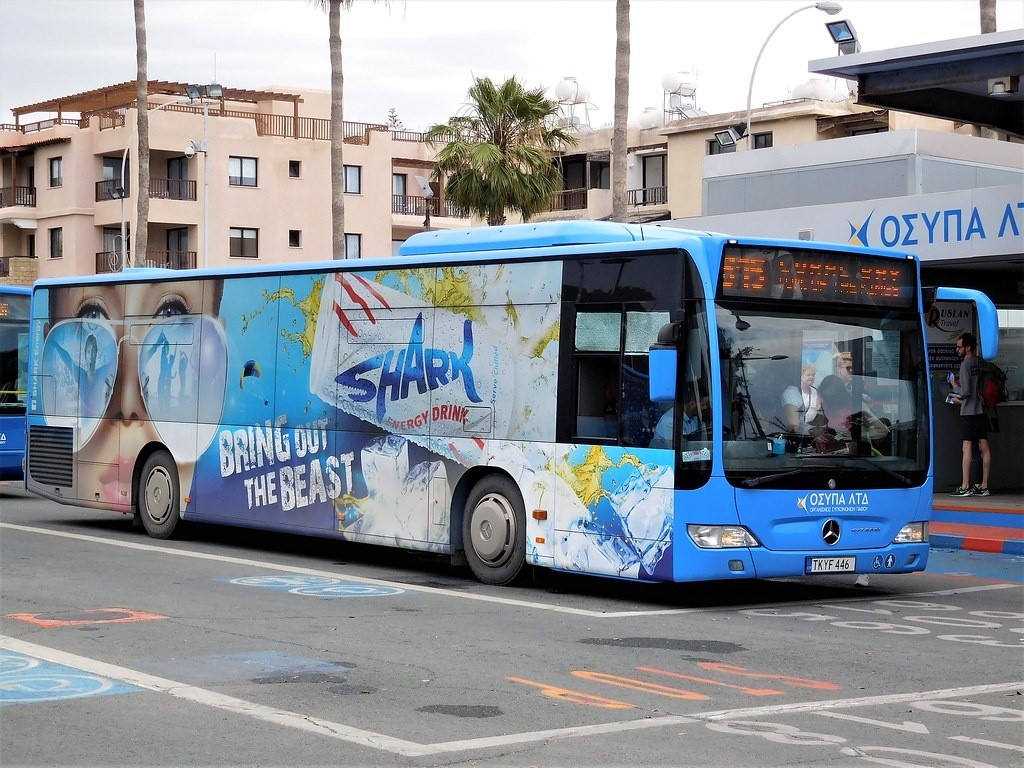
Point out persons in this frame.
[43,276,225,519]
[779,351,872,447]
[948,333,992,496]
[649,389,708,448]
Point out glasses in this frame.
[837,366,853,372]
[40,316,227,464]
[956,346,964,350]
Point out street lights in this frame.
[747,1,844,152]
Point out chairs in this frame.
[622,400,663,448]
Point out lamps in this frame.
[183,84,223,106]
[825,19,861,57]
[107,186,126,200]
[989,81,1014,99]
[715,121,748,150]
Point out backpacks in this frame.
[973,355,1007,406]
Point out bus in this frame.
[0,284,33,481]
[21,211,1000,586]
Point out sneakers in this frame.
[949,487,970,496]
[969,484,990,496]
